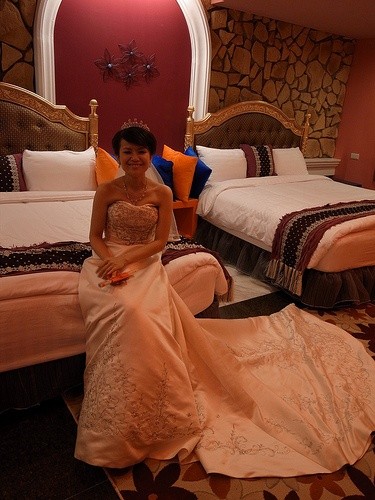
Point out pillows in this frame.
[0,154,25,192]
[96,147,124,187]
[22,145,97,192]
[195,144,247,182]
[272,147,309,176]
[150,144,212,202]
[239,144,278,177]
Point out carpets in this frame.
[60,291,375,500]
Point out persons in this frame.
[74,119,375,478]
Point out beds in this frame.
[184,100,375,310]
[0,81,233,376]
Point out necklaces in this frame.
[123,175,148,205]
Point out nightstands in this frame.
[171,199,199,241]
